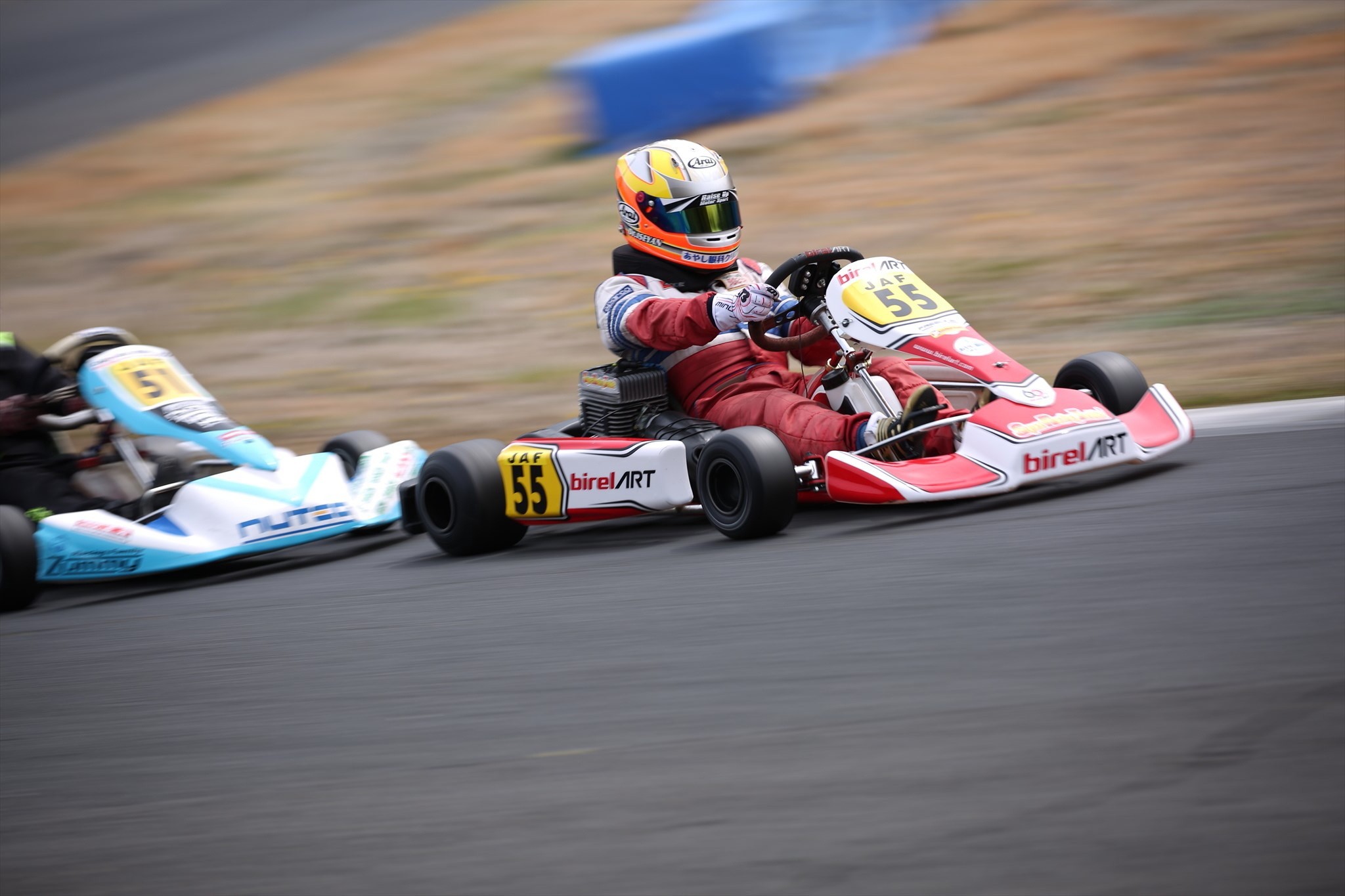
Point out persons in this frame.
[0,330,196,524]
[593,138,997,466]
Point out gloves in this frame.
[712,283,779,332]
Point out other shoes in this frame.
[863,384,939,462]
[971,387,995,414]
[132,455,184,525]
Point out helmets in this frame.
[615,139,743,270]
